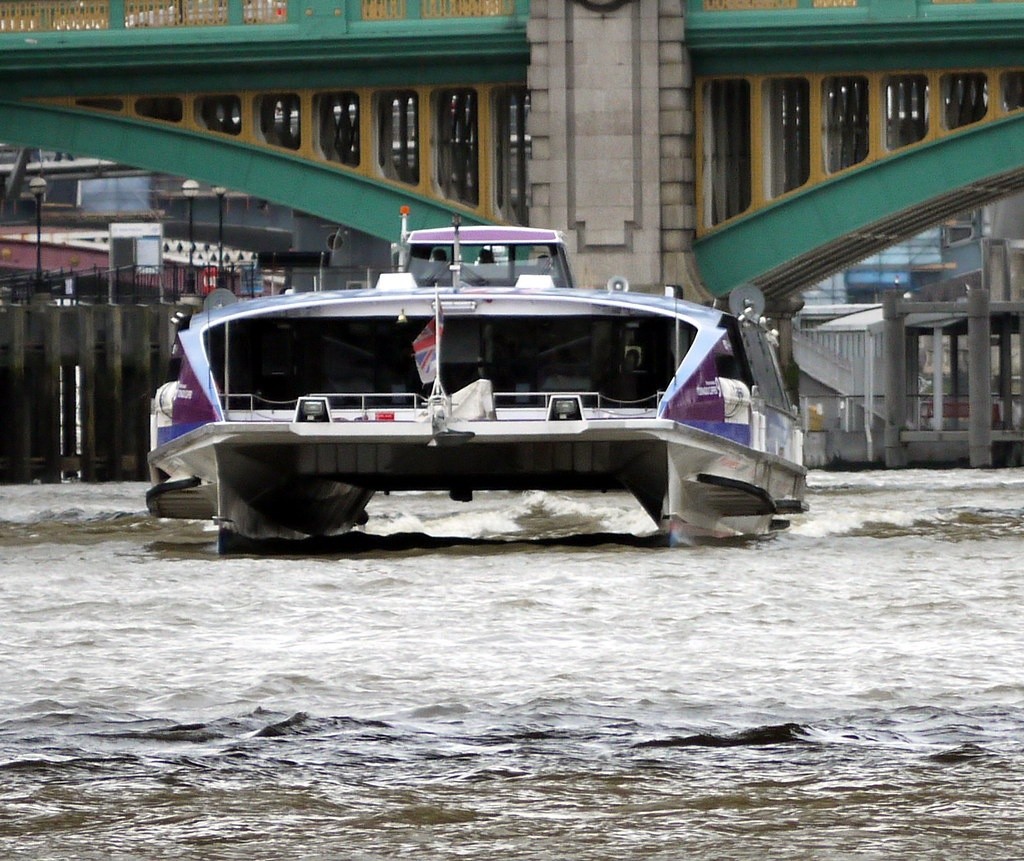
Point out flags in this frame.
[412,305,443,384]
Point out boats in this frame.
[145,205,811,554]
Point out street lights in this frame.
[180,179,200,305]
[29,177,52,307]
[211,184,227,289]
